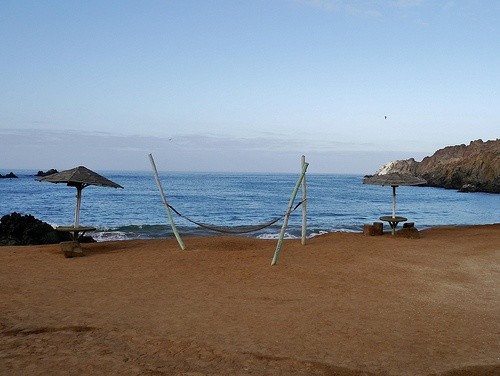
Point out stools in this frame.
[403,223,415,228]
[63,241,83,257]
[364,222,383,236]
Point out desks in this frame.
[56,227,96,232]
[380,216,407,221]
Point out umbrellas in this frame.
[35,166,125,227]
[362,169,427,219]
